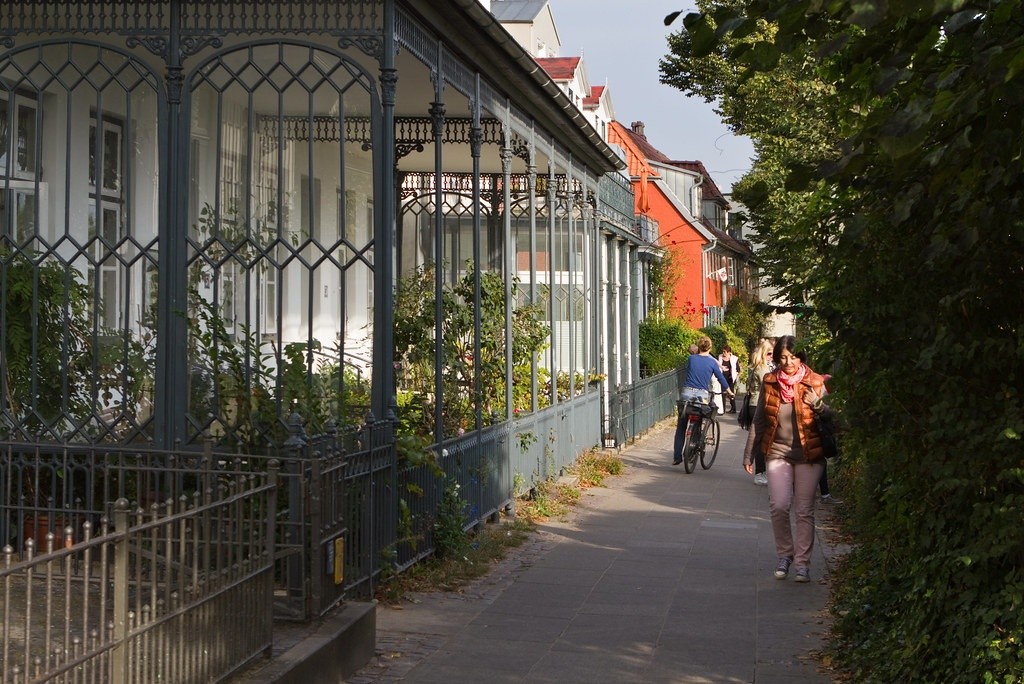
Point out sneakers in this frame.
[794,568,810,581]
[774,555,794,580]
[754,472,768,486]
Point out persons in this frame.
[744,335,833,582]
[672,335,833,499]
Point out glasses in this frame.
[767,353,772,356]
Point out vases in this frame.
[22,515,85,556]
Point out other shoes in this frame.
[821,495,834,504]
[672,460,683,465]
[726,409,736,414]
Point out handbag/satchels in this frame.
[737,391,752,432]
[814,412,839,459]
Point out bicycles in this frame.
[683,389,735,474]
